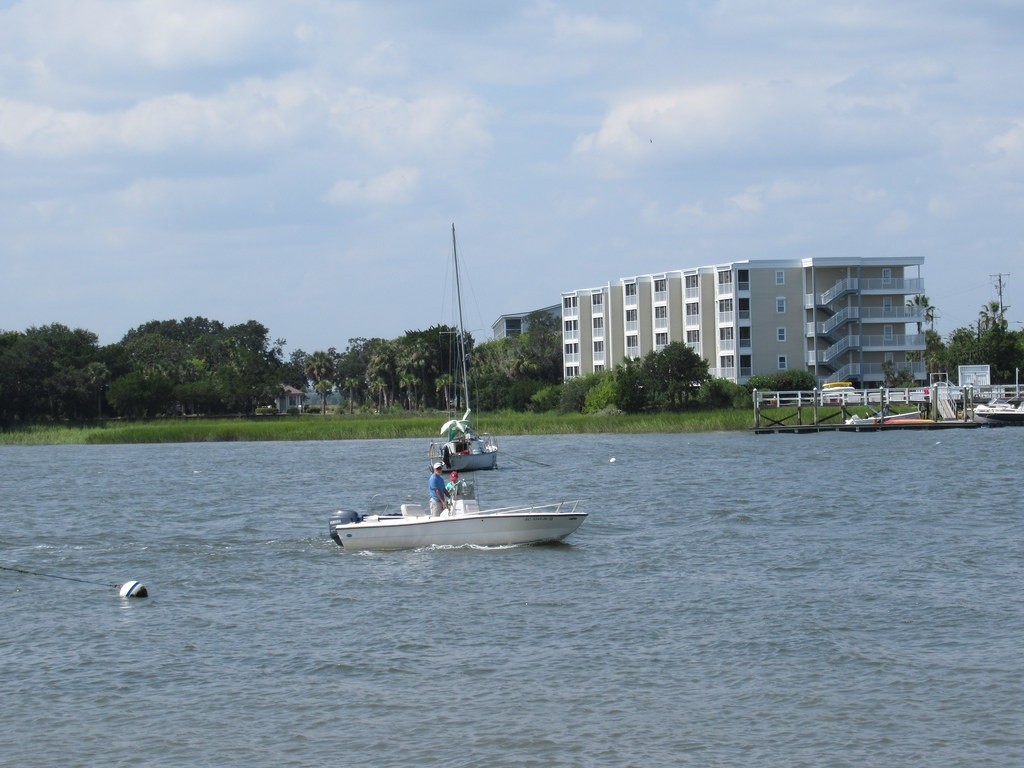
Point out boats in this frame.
[329,474,593,553]
[844,410,923,426]
[973,367,1024,426]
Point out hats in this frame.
[451,471,458,480]
[433,463,442,469]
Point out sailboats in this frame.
[427,222,498,475]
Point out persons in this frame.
[445,471,463,508]
[465,427,471,446]
[428,462,451,517]
